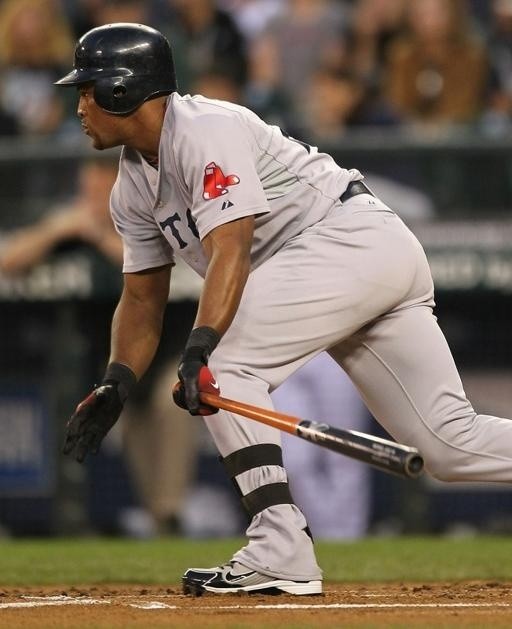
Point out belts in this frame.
[340,181,372,203]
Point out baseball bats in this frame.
[173,380,425,480]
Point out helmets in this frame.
[53,22,177,115]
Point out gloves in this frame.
[61,384,124,462]
[177,347,220,416]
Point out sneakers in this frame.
[183,559,324,595]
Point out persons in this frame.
[54,21,512,596]
[1,0,510,537]
